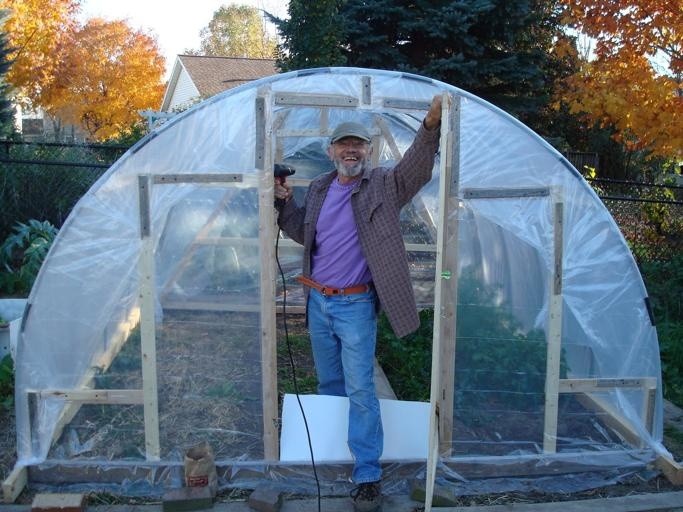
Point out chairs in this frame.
[354,482,382,511]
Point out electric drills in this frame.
[274,163,296,185]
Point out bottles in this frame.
[331,123,370,144]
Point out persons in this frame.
[272,93,455,512]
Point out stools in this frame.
[296,276,366,296]
